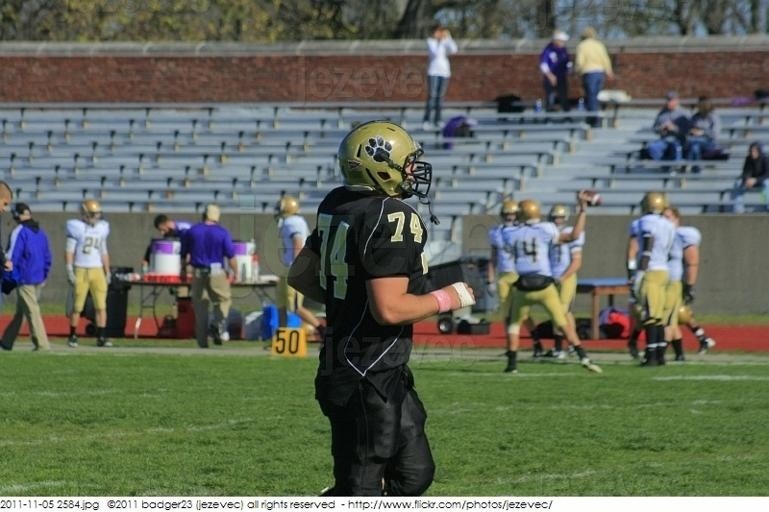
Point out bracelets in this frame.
[431,290,450,314]
[182,266,187,270]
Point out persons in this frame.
[64,199,112,346]
[0,181,13,272]
[624,191,716,367]
[181,205,240,347]
[0,201,52,352]
[422,25,458,132]
[682,95,714,161]
[263,196,326,351]
[573,27,614,111]
[647,90,690,160]
[537,30,573,112]
[288,120,476,497]
[742,141,769,188]
[486,191,603,373]
[155,214,194,319]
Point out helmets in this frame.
[79,200,101,224]
[515,199,543,224]
[276,196,299,218]
[338,119,432,197]
[641,192,666,215]
[549,203,570,223]
[498,198,519,221]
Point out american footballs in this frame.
[577,190,604,207]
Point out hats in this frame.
[203,203,220,223]
[13,203,31,214]
[552,30,570,42]
[666,91,680,101]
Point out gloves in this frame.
[66,265,76,286]
[487,284,497,297]
[105,271,112,285]
[630,269,646,298]
[684,285,696,305]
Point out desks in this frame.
[118,273,280,341]
[574,277,636,340]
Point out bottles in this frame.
[140,260,148,276]
[251,255,262,281]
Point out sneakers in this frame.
[627,341,638,357]
[538,348,567,361]
[208,323,222,346]
[532,343,543,357]
[581,357,604,374]
[697,338,716,355]
[96,337,112,348]
[675,353,685,360]
[568,344,578,357]
[67,333,78,348]
[503,366,518,374]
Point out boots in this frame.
[657,345,666,366]
[641,346,656,366]
